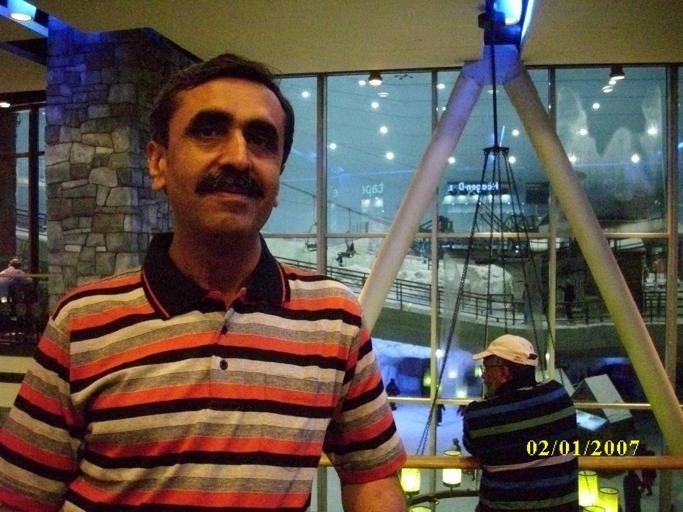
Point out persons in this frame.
[0,258,33,295]
[0,53,412,512]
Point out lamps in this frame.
[400,450,620,512]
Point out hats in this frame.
[472,334,538,366]
